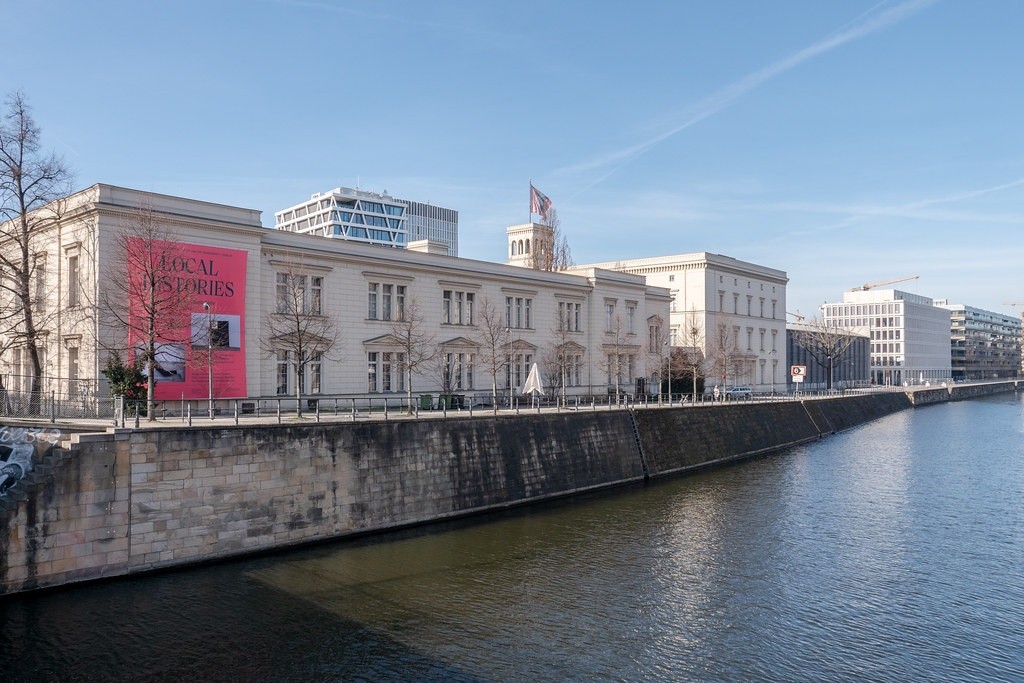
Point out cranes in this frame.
[1002,302,1023,319]
[785,310,804,321]
[849,275,920,291]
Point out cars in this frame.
[722,386,752,398]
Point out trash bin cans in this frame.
[439,393,466,410]
[420,394,432,410]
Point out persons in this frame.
[712,385,720,403]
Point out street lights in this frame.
[663,341,672,405]
[203,302,215,419]
[505,328,514,410]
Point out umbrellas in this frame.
[522,362,545,410]
